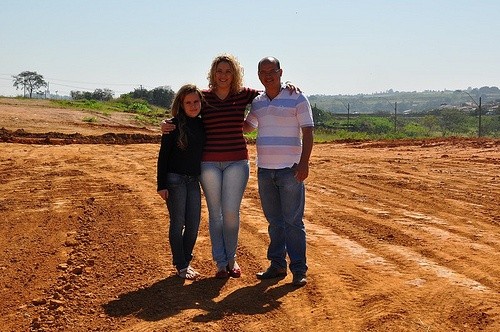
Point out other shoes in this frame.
[228,260,241,277]
[215,266,230,279]
[255,266,287,279]
[293,272,307,286]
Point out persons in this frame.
[244,57,314,285]
[157,85,201,279]
[160,56,303,278]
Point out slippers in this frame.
[175,266,200,279]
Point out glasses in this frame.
[260,69,279,76]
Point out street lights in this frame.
[336,99,349,131]
[377,96,397,133]
[463,91,482,137]
